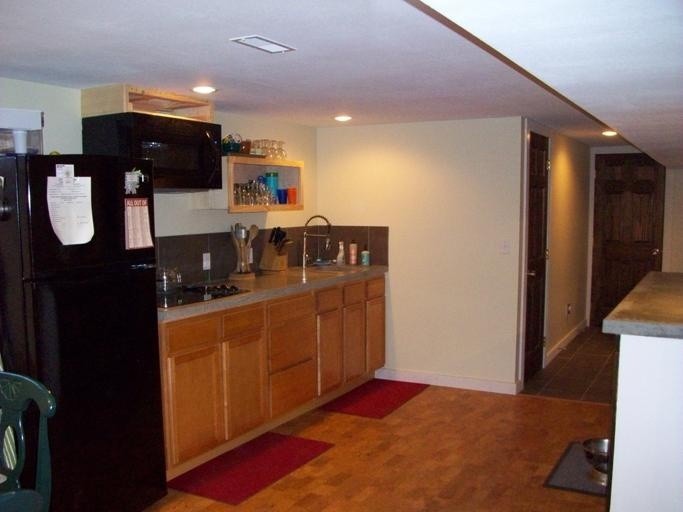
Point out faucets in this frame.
[302,215,331,269]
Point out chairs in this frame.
[0,371,58,512]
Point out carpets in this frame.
[543,441,608,498]
[166,433,335,506]
[320,379,429,420]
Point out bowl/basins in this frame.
[581,435,614,474]
[221,144,242,155]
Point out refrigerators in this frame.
[0,154,168,512]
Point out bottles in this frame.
[235,133,286,206]
[337,239,370,266]
[221,133,243,143]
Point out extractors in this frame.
[82,111,224,189]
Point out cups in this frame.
[287,187,297,205]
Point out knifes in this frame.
[268,227,287,248]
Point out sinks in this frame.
[306,265,351,271]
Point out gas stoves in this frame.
[155,284,251,309]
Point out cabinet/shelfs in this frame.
[316,284,368,396]
[366,274,387,371]
[160,302,266,465]
[209,156,305,213]
[81,84,215,123]
[266,290,318,421]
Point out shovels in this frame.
[246,224,259,248]
